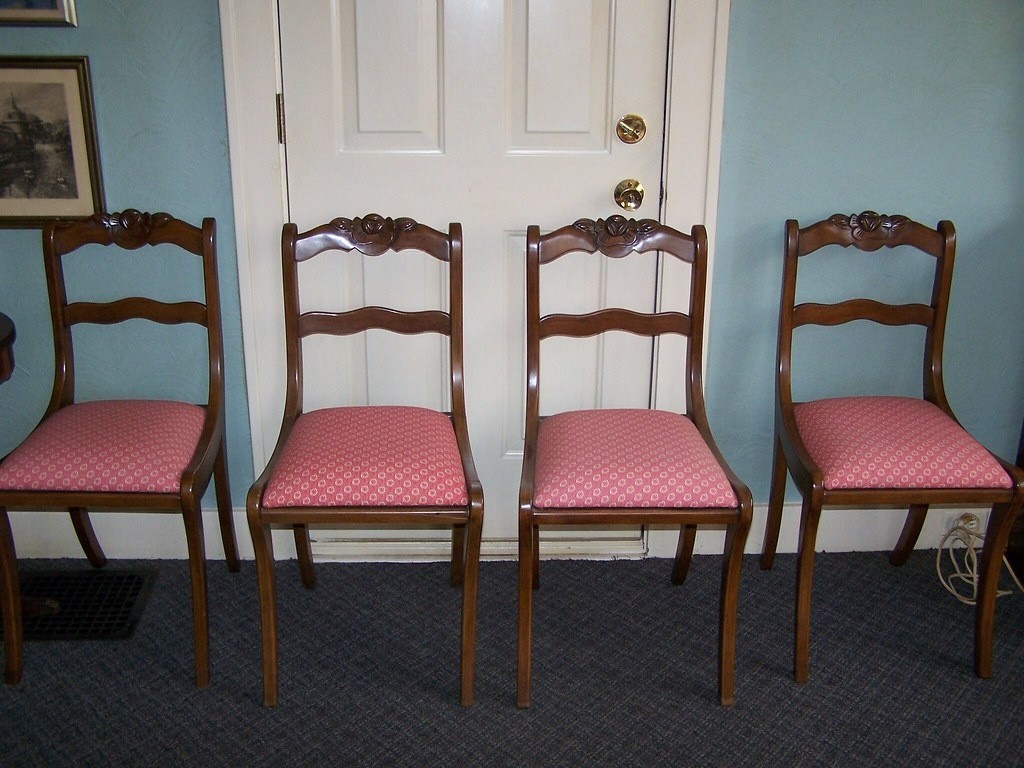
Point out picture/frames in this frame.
[0,0,77,28]
[0,55,107,228]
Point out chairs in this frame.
[760,214,1011,682]
[508,214,754,705]
[243,214,486,710]
[0,208,237,688]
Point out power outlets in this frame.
[938,511,990,539]
